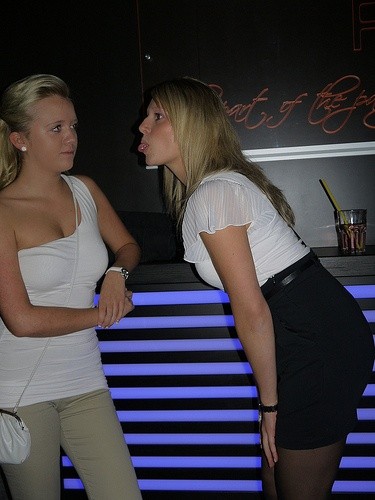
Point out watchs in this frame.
[258,403,280,413]
[106,267,130,279]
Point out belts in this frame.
[264,256,318,301]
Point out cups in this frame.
[337,209,367,256]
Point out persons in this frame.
[137,77,375,500]
[0,73,143,500]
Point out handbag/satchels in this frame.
[108,210,179,265]
[0,409,31,464]
